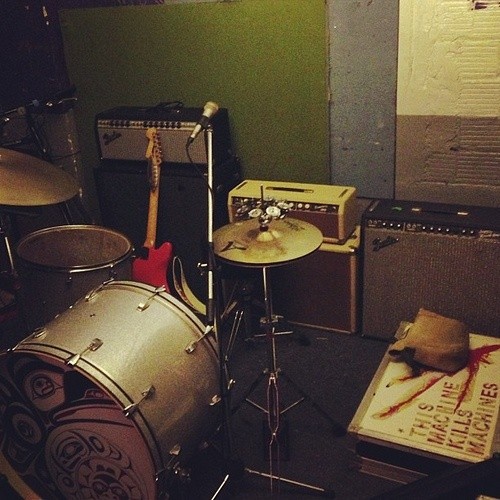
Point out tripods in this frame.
[232,266,333,459]
[210,303,329,500]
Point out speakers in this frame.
[363,201,500,350]
[92,104,238,324]
[223,228,361,336]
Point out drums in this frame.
[11,224,136,337]
[0,281,231,500]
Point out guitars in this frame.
[132,126,173,295]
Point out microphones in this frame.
[186,101,219,145]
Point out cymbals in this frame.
[0,146,80,207]
[211,216,324,268]
